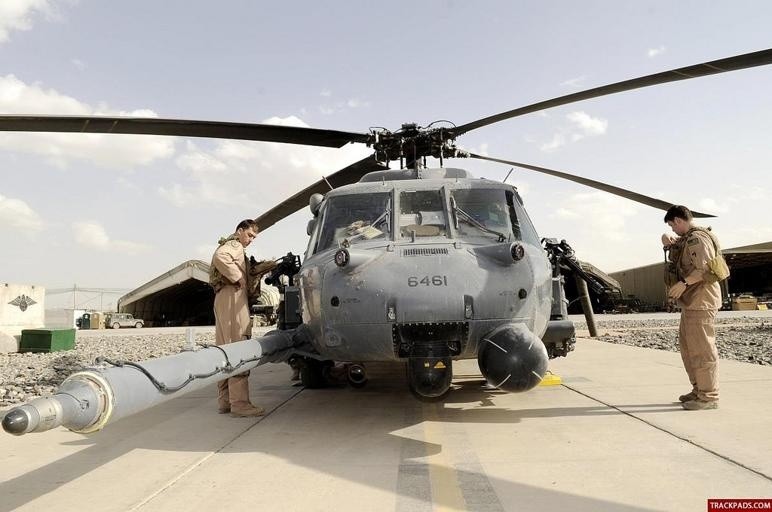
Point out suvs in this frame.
[105,312,145,328]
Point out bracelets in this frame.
[680,277,688,288]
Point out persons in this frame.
[659,204,726,412]
[205,215,280,417]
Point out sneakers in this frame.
[231,404,264,416]
[216,407,230,414]
[679,392,698,401]
[683,398,716,410]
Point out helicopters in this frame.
[1,42,772,439]
